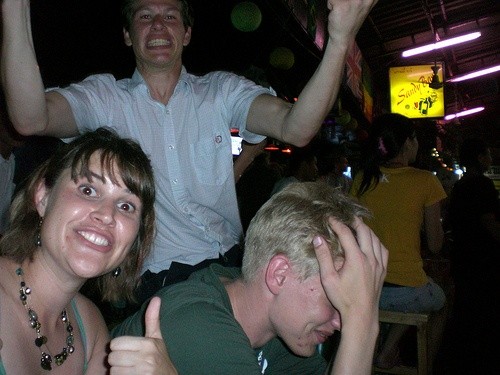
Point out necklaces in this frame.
[16,261,74,372]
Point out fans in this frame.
[407,15,468,89]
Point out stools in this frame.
[378,310,434,375]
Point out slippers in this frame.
[379,350,402,369]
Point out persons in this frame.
[233,113,500,369]
[0,0,379,318]
[110,178,389,375]
[0,126,158,375]
